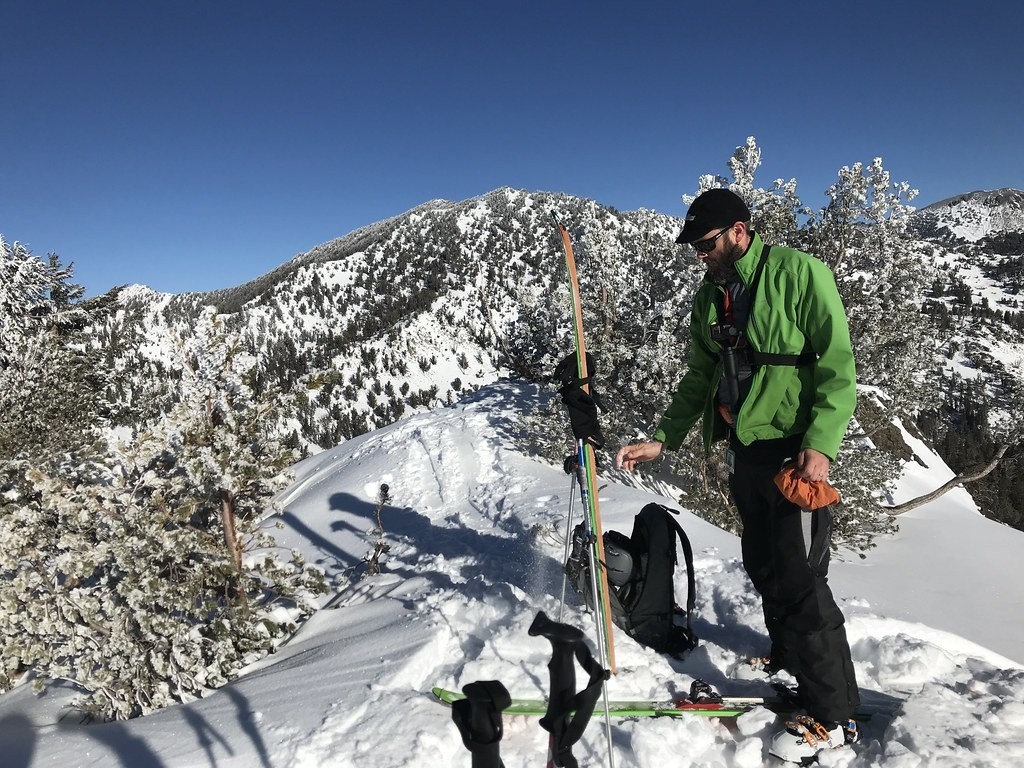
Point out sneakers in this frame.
[769,709,859,765]
[739,654,802,691]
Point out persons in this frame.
[617,188,860,761]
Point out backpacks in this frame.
[565,502,698,656]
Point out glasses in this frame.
[690,226,732,251]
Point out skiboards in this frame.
[433,686,813,718]
[550,208,619,678]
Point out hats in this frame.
[674,188,751,244]
